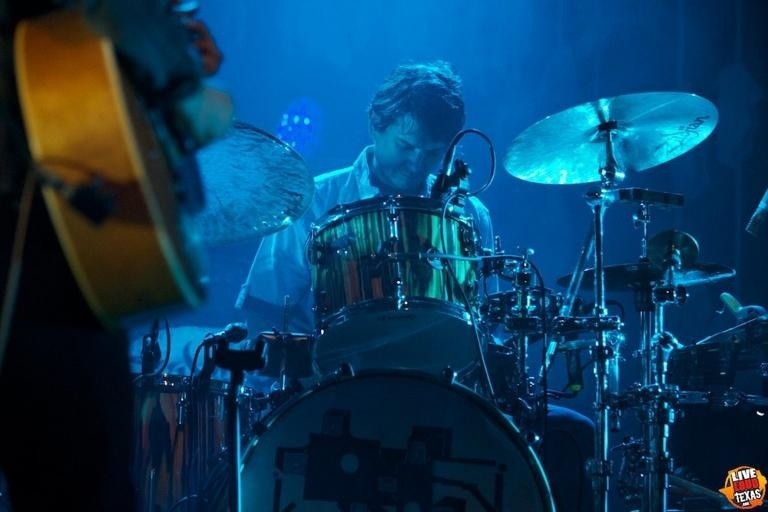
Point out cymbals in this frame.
[557,264,736,289]
[191,120,312,243]
[504,91,720,185]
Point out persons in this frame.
[233,59,598,511]
[1,1,226,510]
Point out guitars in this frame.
[15,1,222,319]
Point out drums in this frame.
[133,374,256,511]
[664,394,767,490]
[237,362,556,510]
[306,196,489,380]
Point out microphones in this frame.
[206,323,249,342]
[431,138,458,201]
